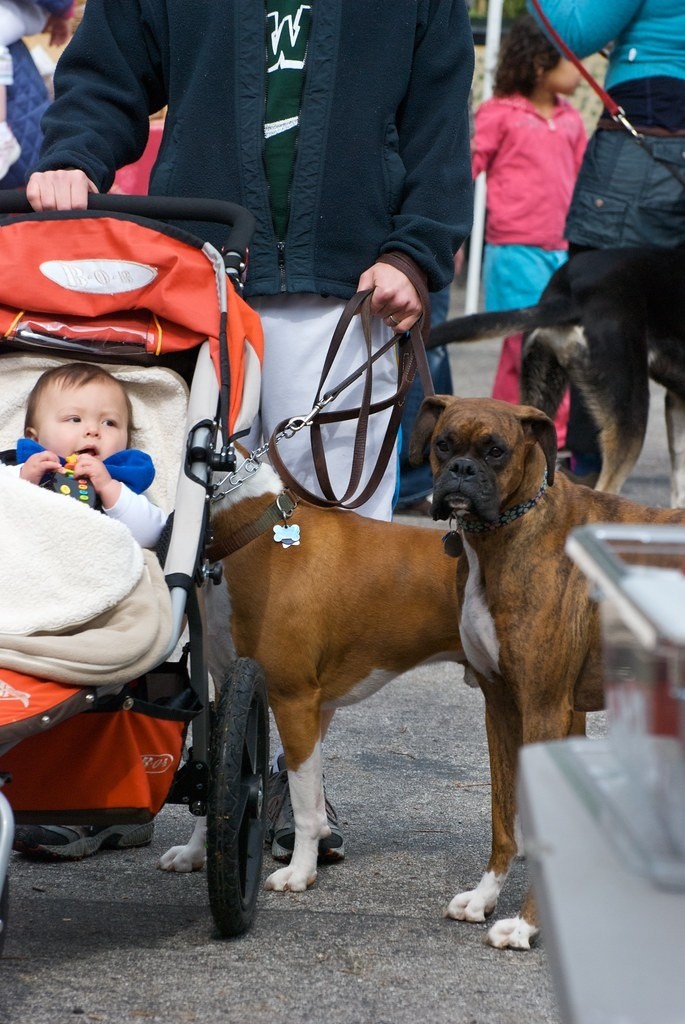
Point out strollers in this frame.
[0,180,274,942]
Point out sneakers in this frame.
[263,752,346,865]
[11,822,156,861]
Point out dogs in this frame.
[406,392,685,948]
[157,427,481,893]
[401,242,685,509]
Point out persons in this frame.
[0,0,86,191]
[5,0,475,861]
[525,0,685,485]
[464,11,588,451]
[0,362,166,547]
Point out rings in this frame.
[389,316,398,325]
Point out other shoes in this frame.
[0,123,22,182]
